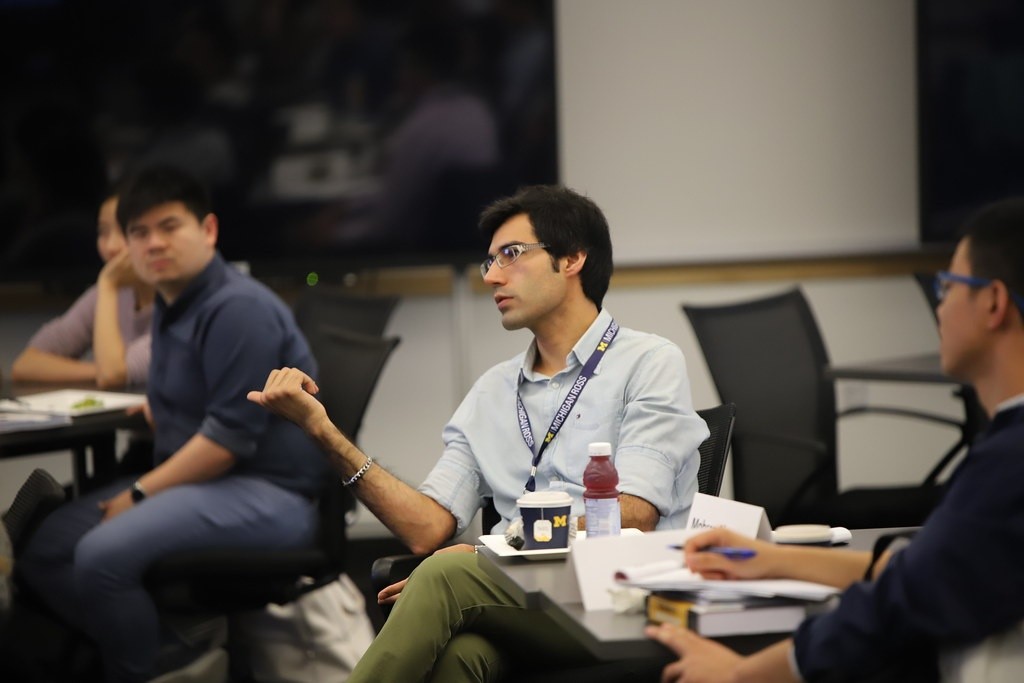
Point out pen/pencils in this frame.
[666,544,754,560]
[7,396,32,408]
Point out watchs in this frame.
[128,481,145,505]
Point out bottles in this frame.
[583,443,622,538]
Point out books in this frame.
[644,589,806,637]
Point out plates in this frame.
[478,528,644,561]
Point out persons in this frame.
[16,167,321,682]
[248,183,710,683]
[8,195,153,389]
[644,201,1023,683]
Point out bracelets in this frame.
[340,456,373,488]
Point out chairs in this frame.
[682,284,969,524]
[291,291,404,346]
[133,324,405,683]
[383,401,738,631]
[916,266,942,327]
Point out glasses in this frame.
[934,269,1024,318]
[479,243,548,277]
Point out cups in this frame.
[516,491,574,550]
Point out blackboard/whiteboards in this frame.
[0,1,1022,322]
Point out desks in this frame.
[0,367,149,490]
[477,525,926,662]
[827,352,964,385]
